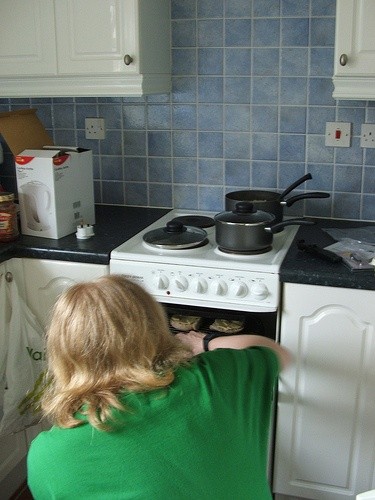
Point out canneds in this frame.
[0,193,20,241]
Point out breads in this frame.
[170,315,202,330]
[208,319,244,334]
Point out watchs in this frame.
[202,332,222,353]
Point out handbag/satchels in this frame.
[0,274,47,435]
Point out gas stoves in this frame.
[109,207,303,274]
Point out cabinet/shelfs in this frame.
[271,282,375,500]
[331,0,374,101]
[0,257,108,500]
[0,0,173,97]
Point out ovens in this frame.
[109,259,281,487]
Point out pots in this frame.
[224,190,330,234]
[214,202,318,252]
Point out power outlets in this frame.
[360,124,375,148]
[85,117,105,139]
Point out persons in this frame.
[27,275,292,500]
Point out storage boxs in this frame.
[0,108,96,240]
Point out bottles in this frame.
[0,191,19,241]
